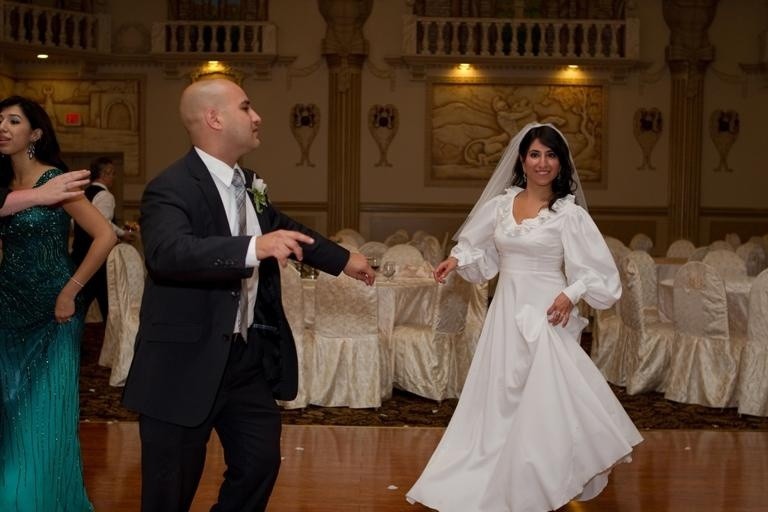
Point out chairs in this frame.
[97,242,146,389]
[408,227,446,264]
[376,225,410,246]
[750,235,768,249]
[590,232,632,367]
[663,261,748,409]
[308,242,394,410]
[725,231,741,247]
[358,239,390,268]
[708,240,736,253]
[599,249,673,388]
[326,227,365,251]
[464,279,489,362]
[315,241,362,279]
[629,233,653,250]
[666,239,696,259]
[275,259,314,410]
[389,269,472,409]
[701,248,748,278]
[736,267,768,418]
[376,243,437,277]
[735,241,765,273]
[687,247,710,262]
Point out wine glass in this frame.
[383,264,394,284]
[386,261,396,282]
[370,256,381,271]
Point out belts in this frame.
[232,332,251,344]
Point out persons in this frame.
[0,169,90,217]
[0,96,118,512]
[119,78,376,512]
[72,155,137,333]
[405,121,645,512]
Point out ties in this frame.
[231,170,253,345]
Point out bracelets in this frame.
[69,278,84,289]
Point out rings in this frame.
[553,310,561,320]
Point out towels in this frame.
[416,259,436,278]
